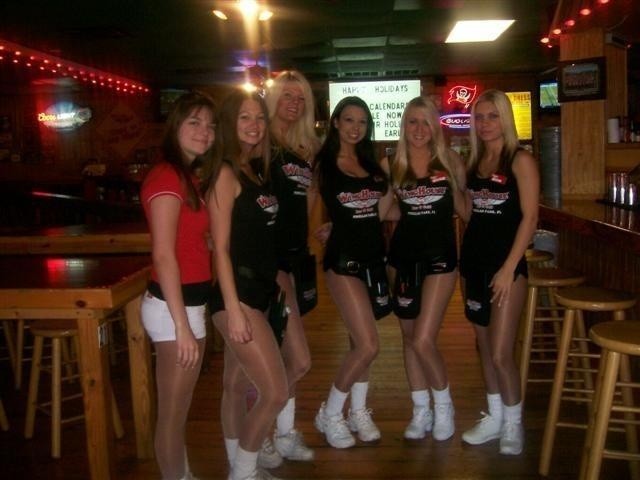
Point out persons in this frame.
[140,93,224,480]
[258,71,323,470]
[314,96,467,442]
[207,86,289,480]
[310,96,390,448]
[459,91,540,455]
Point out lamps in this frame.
[541,0,610,43]
[212,8,231,22]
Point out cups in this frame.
[607,118,620,144]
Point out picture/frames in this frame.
[504,90,535,143]
[557,56,606,102]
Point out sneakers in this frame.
[273,429,313,461]
[313,401,355,449]
[403,405,433,439]
[499,421,524,456]
[431,401,456,441]
[227,435,283,480]
[346,407,381,442]
[462,410,502,445]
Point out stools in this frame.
[538,288,640,479]
[579,319,639,480]
[525,248,561,352]
[514,268,595,410]
[24,319,124,459]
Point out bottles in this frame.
[607,170,636,207]
[622,115,637,143]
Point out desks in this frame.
[0,223,152,253]
[1,255,157,461]
